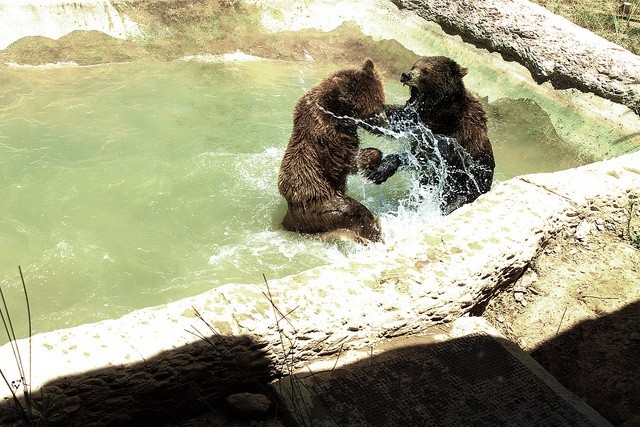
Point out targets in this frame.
[366,55,496,217]
[275,57,389,248]
